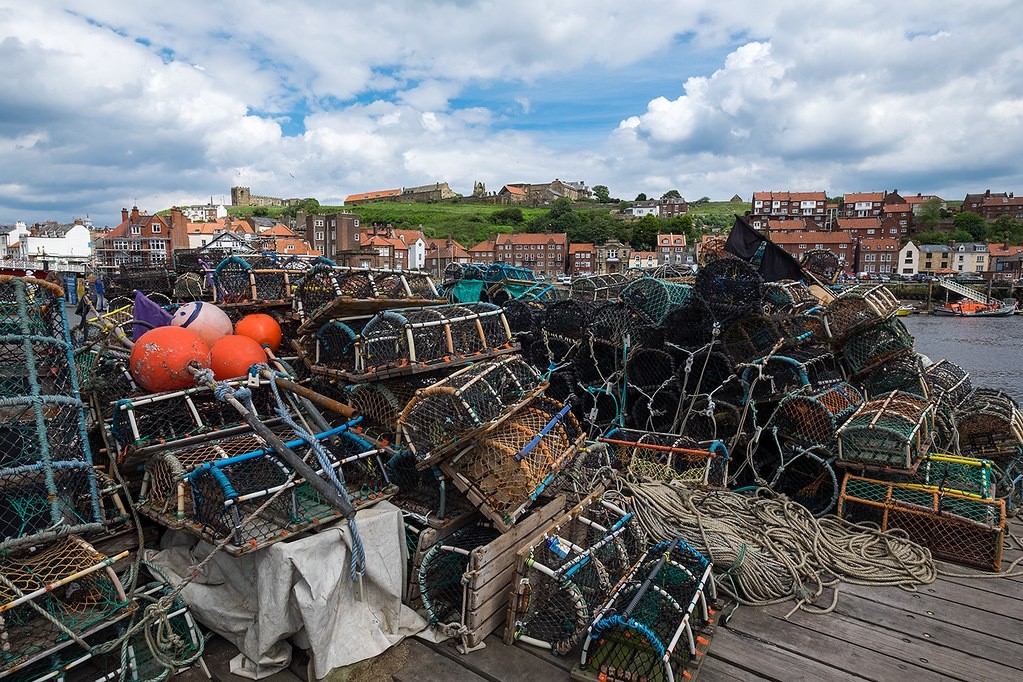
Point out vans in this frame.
[856,272,867,280]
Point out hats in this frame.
[26,271,33,275]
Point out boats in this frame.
[930,298,1018,317]
[895,309,910,315]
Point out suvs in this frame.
[889,274,900,280]
[913,274,926,280]
[900,275,909,280]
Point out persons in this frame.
[95,275,108,312]
[21,270,38,301]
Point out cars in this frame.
[845,273,856,280]
[878,274,890,280]
[935,276,944,281]
[867,274,873,280]
[870,274,878,280]
[929,276,938,281]
[838,275,844,280]
